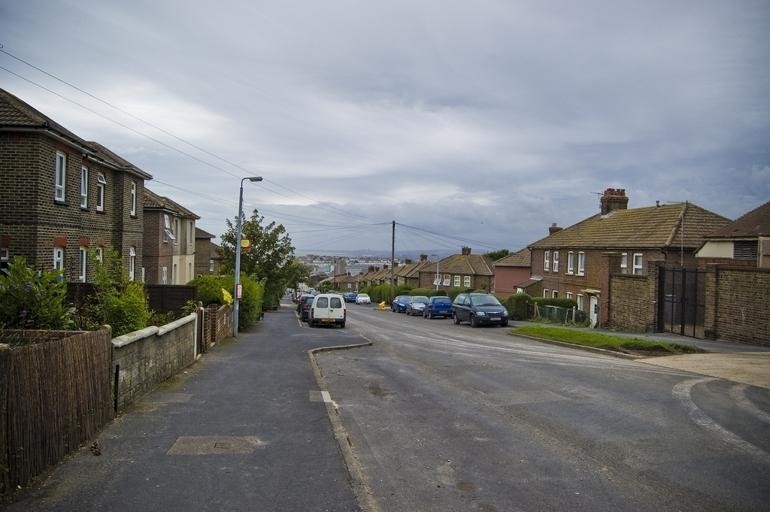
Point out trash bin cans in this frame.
[378,302,385,309]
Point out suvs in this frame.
[450,292,510,330]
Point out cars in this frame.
[424,297,454,321]
[288,287,372,331]
[391,294,412,314]
[405,295,431,317]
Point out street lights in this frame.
[232,176,267,341]
[430,253,439,292]
